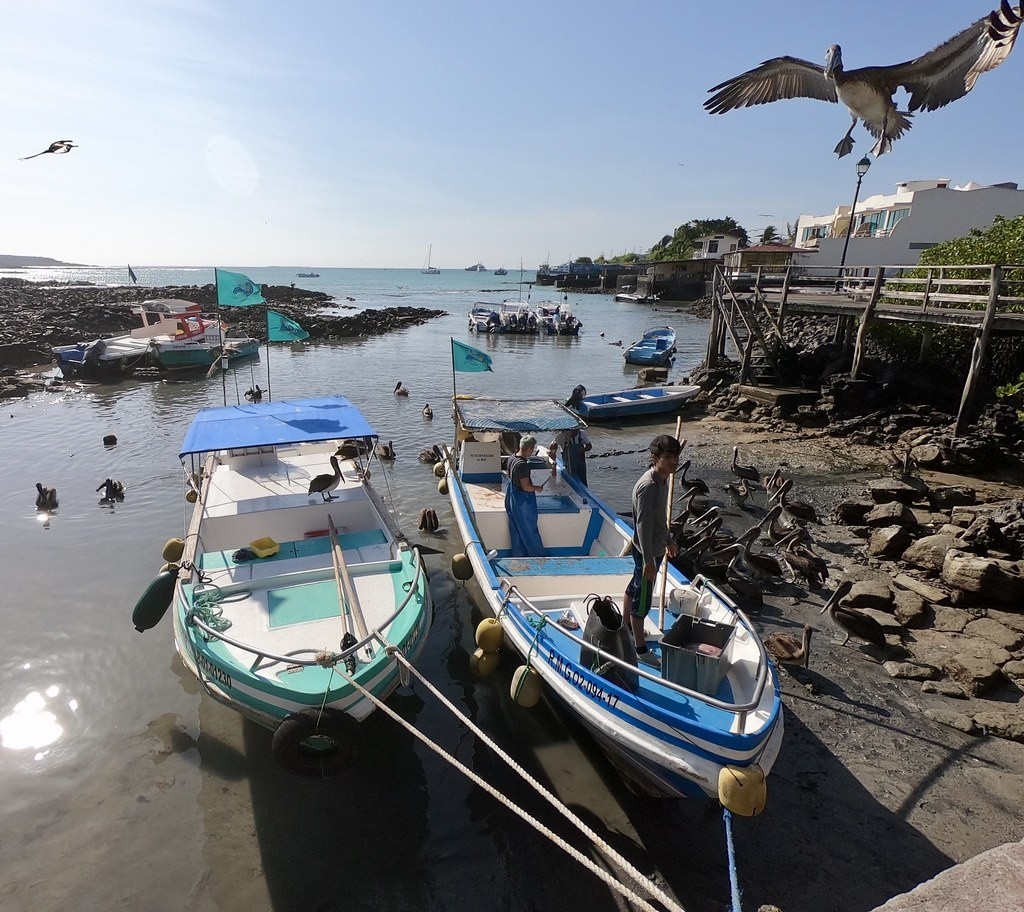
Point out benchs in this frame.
[584,401,598,406]
[637,394,654,399]
[611,397,631,402]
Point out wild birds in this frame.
[18,140,78,162]
[774,526,827,587]
[308,456,345,503]
[422,402,434,419]
[378,441,396,460]
[609,340,622,346]
[336,435,373,461]
[703,0,1024,159]
[736,527,784,593]
[36,482,58,508]
[97,479,126,498]
[712,542,764,607]
[820,580,887,649]
[724,445,822,526]
[758,507,800,544]
[764,624,819,671]
[600,332,605,336]
[672,459,735,570]
[419,445,444,463]
[394,381,409,396]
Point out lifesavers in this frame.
[270,707,366,779]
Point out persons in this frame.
[504,435,548,557]
[623,434,681,669]
[550,429,592,488]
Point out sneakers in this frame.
[636,651,661,669]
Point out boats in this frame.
[432,396,789,818]
[495,268,507,275]
[297,273,320,277]
[517,269,527,272]
[623,326,679,366]
[51,296,261,374]
[168,400,434,751]
[617,293,660,303]
[566,383,702,422]
[467,297,581,335]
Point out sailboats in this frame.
[422,243,441,274]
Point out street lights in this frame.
[834,153,873,291]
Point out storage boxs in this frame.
[658,612,738,696]
[457,440,503,485]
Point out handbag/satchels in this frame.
[584,592,639,692]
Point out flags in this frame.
[216,268,267,306]
[128,266,137,284]
[267,310,309,341]
[452,339,493,372]
